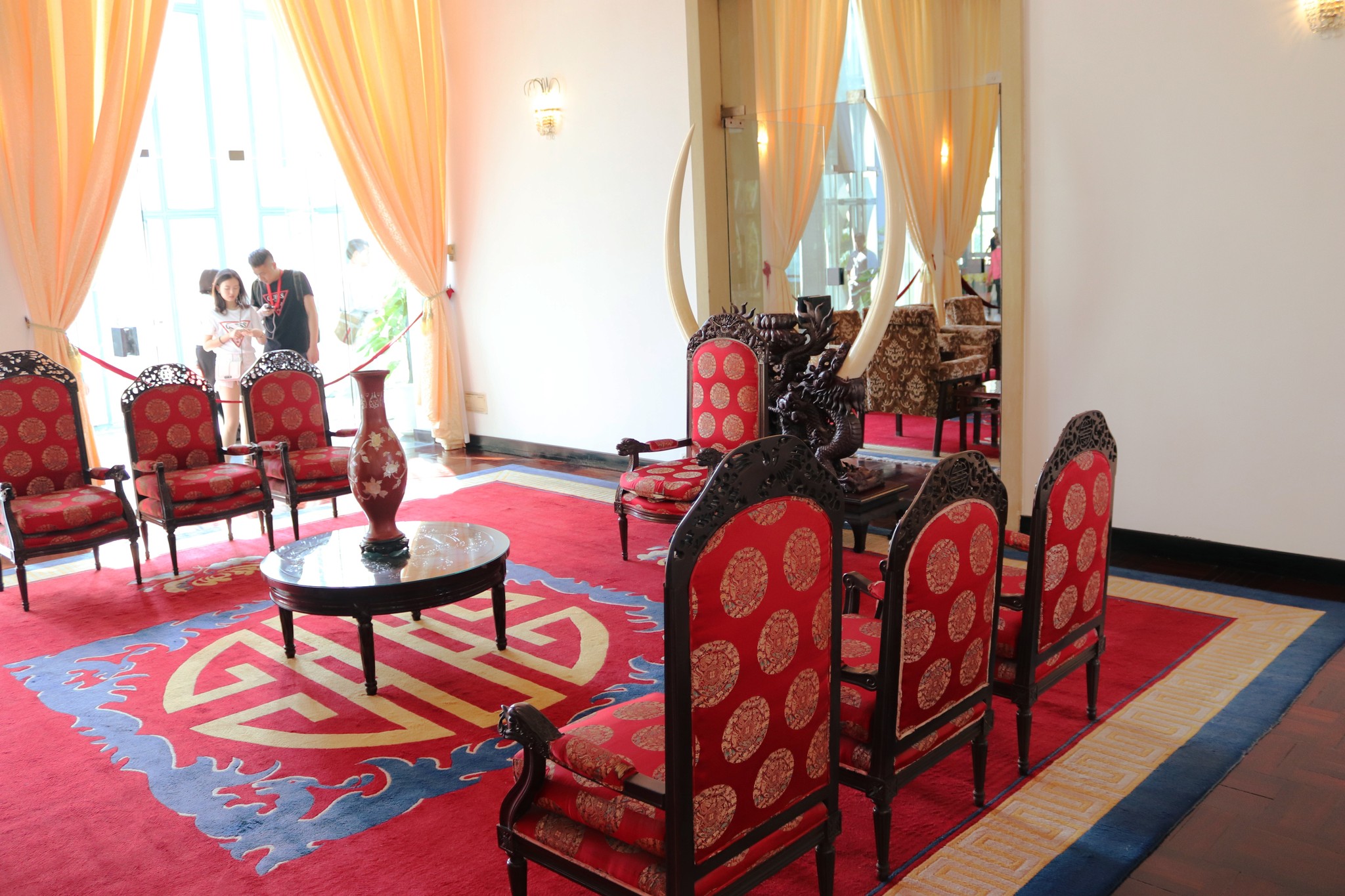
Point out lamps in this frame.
[523,77,565,138]
[1300,0,1345,41]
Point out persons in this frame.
[839,232,879,314]
[986,225,1001,318]
[201,269,267,465]
[248,248,321,371]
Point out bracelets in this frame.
[218,335,225,345]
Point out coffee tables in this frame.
[958,377,1001,452]
[259,519,511,697]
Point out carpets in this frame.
[2,463,1344,896]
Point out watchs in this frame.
[257,332,264,340]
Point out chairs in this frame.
[826,309,863,349]
[613,313,772,562]
[0,350,143,611]
[495,429,848,896]
[900,303,995,383]
[120,363,276,575]
[236,348,359,542]
[943,295,1001,369]
[872,409,1119,778]
[862,305,989,436]
[837,449,1012,884]
